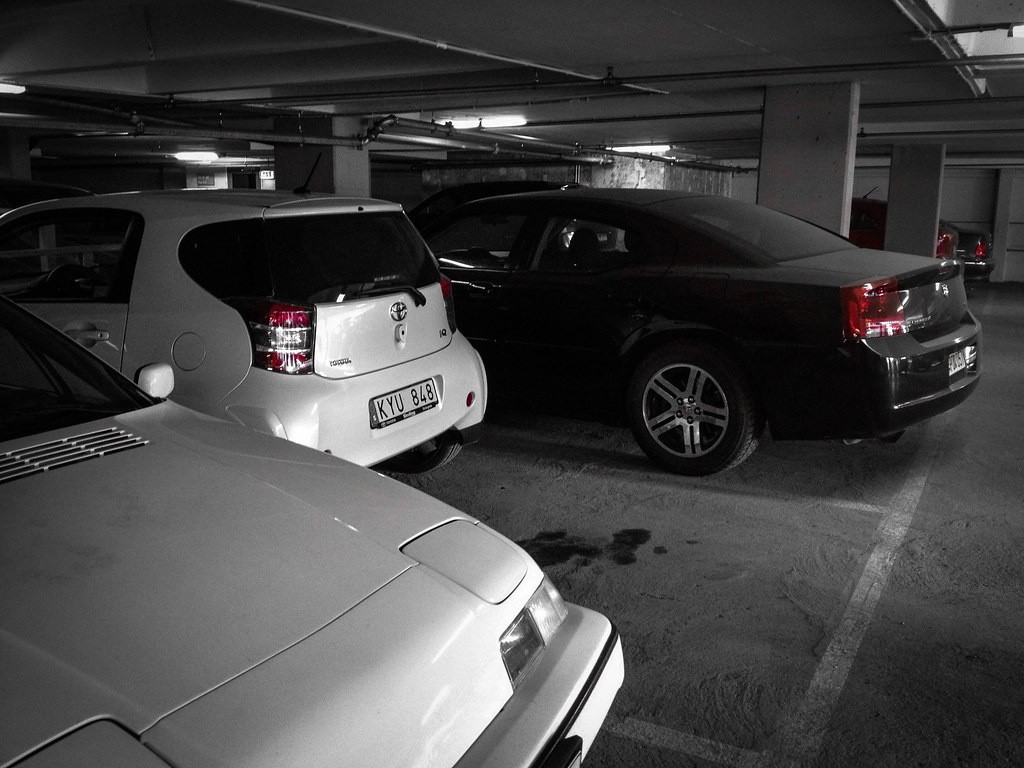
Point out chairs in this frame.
[81,216,648,295]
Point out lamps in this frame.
[605,145,671,152]
[0,83,26,94]
[452,118,528,129]
[175,151,218,160]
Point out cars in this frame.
[421,188,984,478]
[850,197,959,262]
[940,220,996,282]
[405,180,625,253]
[0,295,625,768]
[1,188,489,472]
